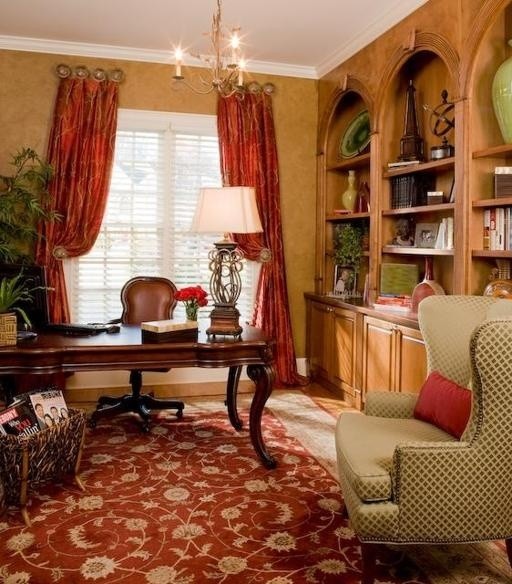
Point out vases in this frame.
[490,36,512,143]
[411,255,446,310]
[187,313,202,332]
[483,257,511,296]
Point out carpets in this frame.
[0,388,512,584]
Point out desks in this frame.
[0,326,279,472]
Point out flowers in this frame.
[174,285,210,320]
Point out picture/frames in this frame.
[333,265,356,297]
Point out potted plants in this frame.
[0,269,44,345]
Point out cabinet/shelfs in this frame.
[304,293,359,408]
[373,31,459,312]
[459,0,512,296]
[359,312,429,410]
[316,76,373,303]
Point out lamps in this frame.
[172,0,248,101]
[188,181,264,342]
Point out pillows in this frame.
[414,371,472,439]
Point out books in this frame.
[434,217,454,251]
[13,388,45,414]
[0,399,39,439]
[481,206,512,252]
[28,390,70,430]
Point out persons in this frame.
[35,404,47,429]
[337,269,351,291]
[50,407,62,425]
[44,414,54,428]
[60,408,69,421]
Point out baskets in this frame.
[0,408,86,499]
[0,315,18,347]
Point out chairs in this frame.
[334,295,512,583]
[85,276,186,428]
